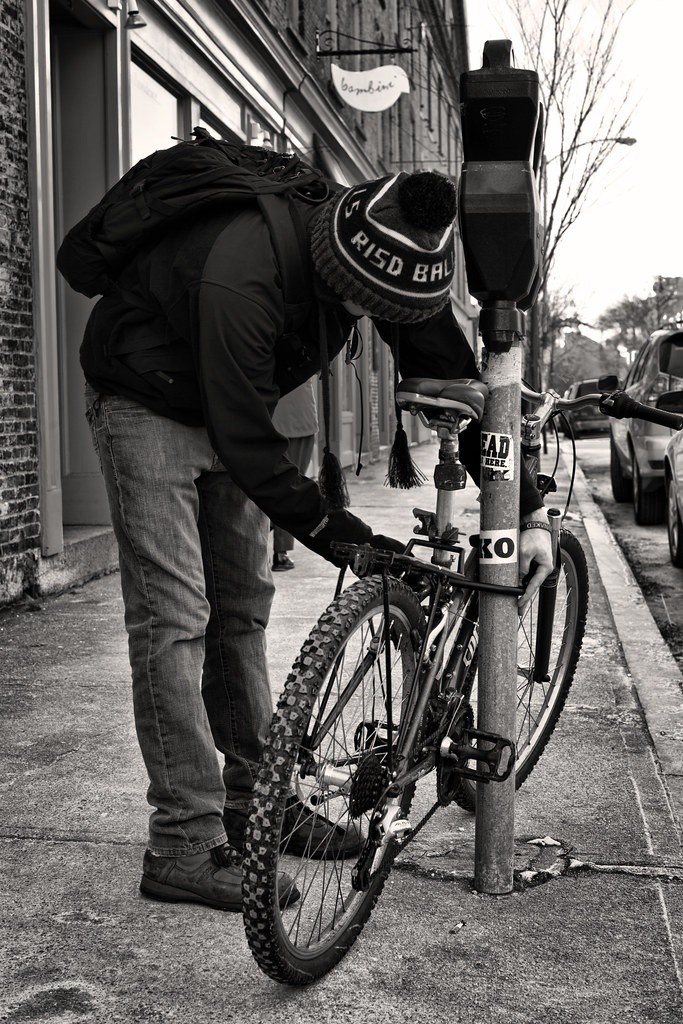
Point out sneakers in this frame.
[139,843,300,910]
[222,796,364,860]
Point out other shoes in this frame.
[271,555,294,571]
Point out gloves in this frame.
[348,535,425,590]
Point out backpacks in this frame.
[57,128,330,357]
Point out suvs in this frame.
[597,318,683,526]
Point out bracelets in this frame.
[520,521,553,533]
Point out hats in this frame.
[309,170,457,324]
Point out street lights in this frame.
[530,135,637,411]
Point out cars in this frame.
[654,389,682,567]
[552,378,623,440]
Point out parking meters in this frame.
[458,41,546,896]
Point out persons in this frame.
[80,162,555,914]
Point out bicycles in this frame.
[241,376,682,986]
